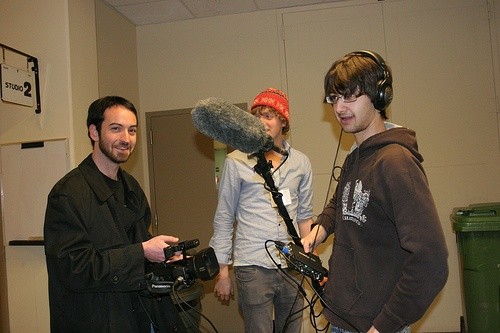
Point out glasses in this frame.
[326,93,363,103]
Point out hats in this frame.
[250,89,290,122]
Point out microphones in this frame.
[190,98,289,156]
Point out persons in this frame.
[209,87,328,333]
[300,50,450,333]
[44,96,192,333]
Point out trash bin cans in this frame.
[449,200,500,333]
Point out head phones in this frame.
[351,50,393,112]
